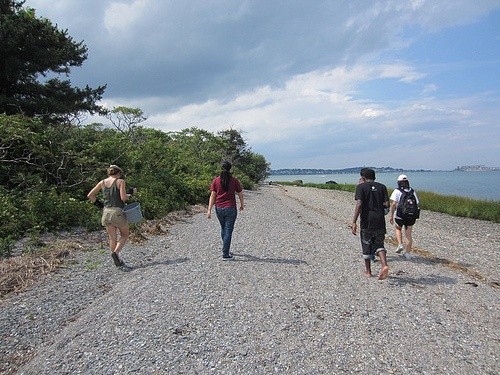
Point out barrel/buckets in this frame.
[122,202,143,224]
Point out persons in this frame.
[352,168,391,280]
[388,173,421,257]
[207,162,245,258]
[87,165,134,268]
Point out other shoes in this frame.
[402,252,411,259]
[395,244,404,253]
[111,252,125,267]
[221,254,234,261]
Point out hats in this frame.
[110,165,123,174]
[396,174,409,181]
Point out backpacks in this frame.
[398,187,420,222]
